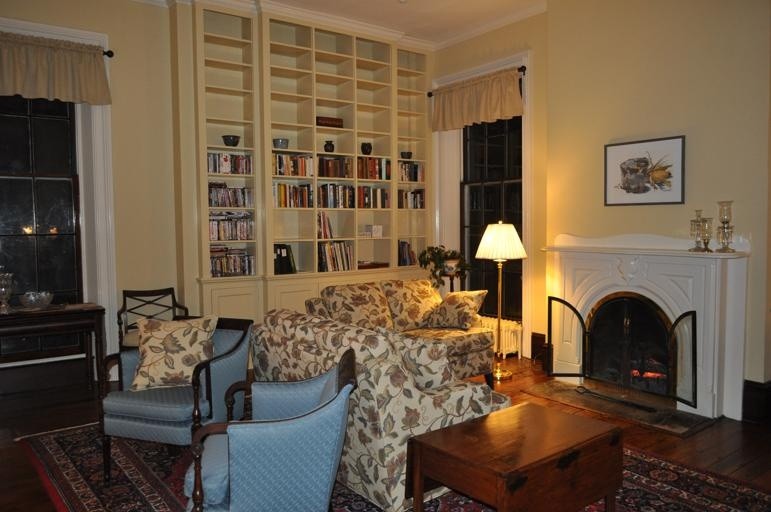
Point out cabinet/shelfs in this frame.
[197,8,432,278]
[0,303,106,423]
[198,266,436,325]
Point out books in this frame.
[398,160,425,183]
[209,181,253,206]
[357,155,390,180]
[398,239,416,266]
[398,187,424,208]
[209,211,254,241]
[211,243,255,277]
[273,151,355,273]
[358,184,391,209]
[207,150,253,175]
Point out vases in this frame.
[324,141,334,152]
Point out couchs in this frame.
[250,308,510,512]
[305,279,495,390]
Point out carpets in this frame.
[1,422,771,512]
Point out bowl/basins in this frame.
[221,135,241,146]
[273,139,289,149]
[400,152,412,159]
[17,294,54,311]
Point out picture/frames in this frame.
[603,135,686,207]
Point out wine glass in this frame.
[0,273,15,314]
[688,200,736,253]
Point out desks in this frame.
[410,399,624,512]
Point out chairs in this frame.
[99,315,254,488]
[184,349,358,512]
[117,287,189,352]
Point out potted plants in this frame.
[418,245,478,289]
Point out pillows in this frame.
[128,314,219,392]
[319,280,488,332]
[373,326,456,392]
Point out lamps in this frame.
[476,220,528,382]
